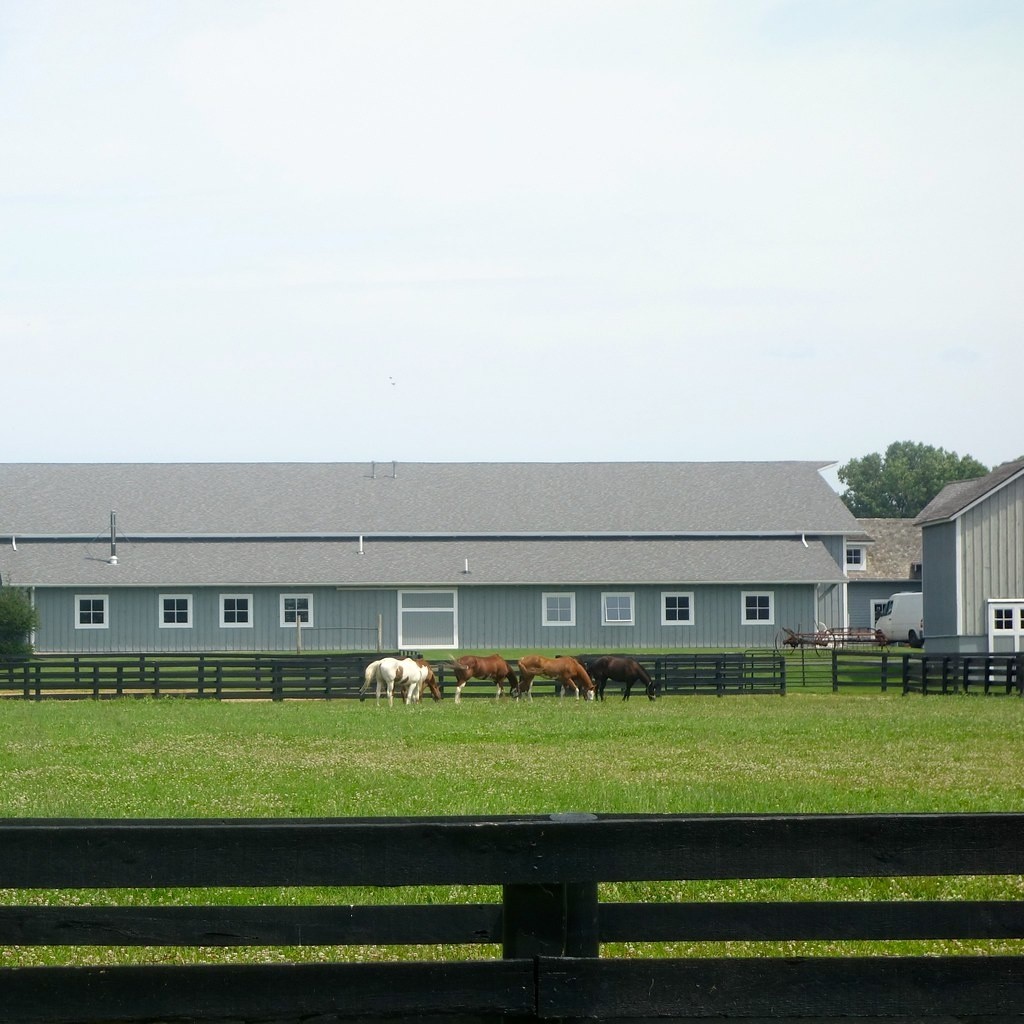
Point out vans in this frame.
[875,592,924,648]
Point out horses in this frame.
[516,655,598,703]
[584,655,657,703]
[359,655,442,708]
[440,653,521,705]
